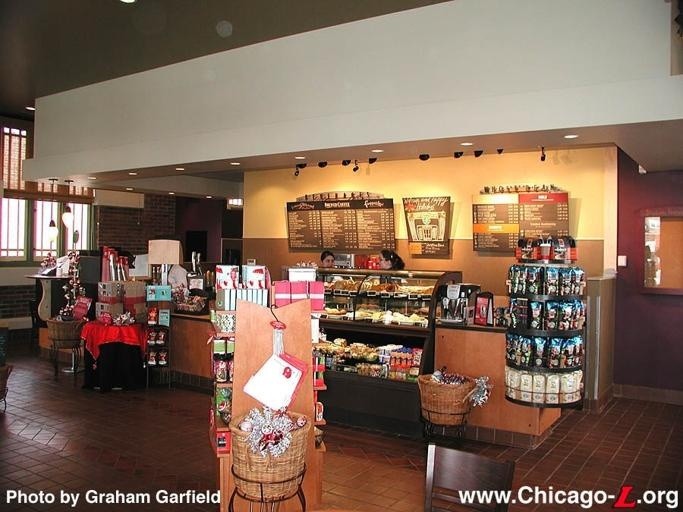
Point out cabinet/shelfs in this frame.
[282,264,462,443]
[204,301,325,511]
[499,261,590,412]
[142,284,173,391]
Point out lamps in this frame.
[46,178,74,244]
[294,164,299,176]
[352,159,360,172]
[539,146,545,163]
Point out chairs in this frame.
[418,440,518,511]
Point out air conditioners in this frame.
[91,188,145,211]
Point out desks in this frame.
[81,321,144,395]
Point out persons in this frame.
[317,251,343,283]
[379,249,404,286]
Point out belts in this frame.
[417,373,477,426]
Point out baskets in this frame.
[228,409,311,498]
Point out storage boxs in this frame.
[94,280,146,323]
